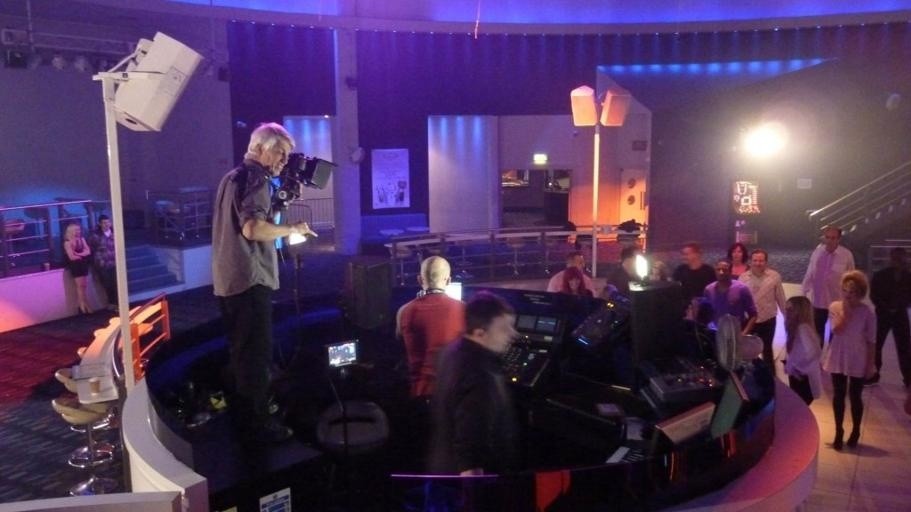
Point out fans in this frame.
[713,312,767,394]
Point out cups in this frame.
[88,377,102,394]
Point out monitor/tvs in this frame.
[707,370,749,443]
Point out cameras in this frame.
[274,151,338,208]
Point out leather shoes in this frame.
[259,421,293,443]
[848,429,859,447]
[833,429,844,450]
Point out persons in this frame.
[207,120,320,442]
[391,253,467,423]
[799,223,857,347]
[819,269,878,450]
[87,213,120,313]
[780,294,822,407]
[425,289,522,511]
[542,241,788,377]
[61,222,93,315]
[862,246,911,388]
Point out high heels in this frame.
[85,308,94,317]
[79,308,88,317]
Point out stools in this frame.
[376,223,598,287]
[50,300,163,496]
[144,184,211,245]
[315,400,390,493]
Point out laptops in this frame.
[320,338,361,384]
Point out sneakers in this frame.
[863,373,880,386]
[903,377,911,386]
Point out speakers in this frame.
[217,67,233,82]
[4,49,27,69]
